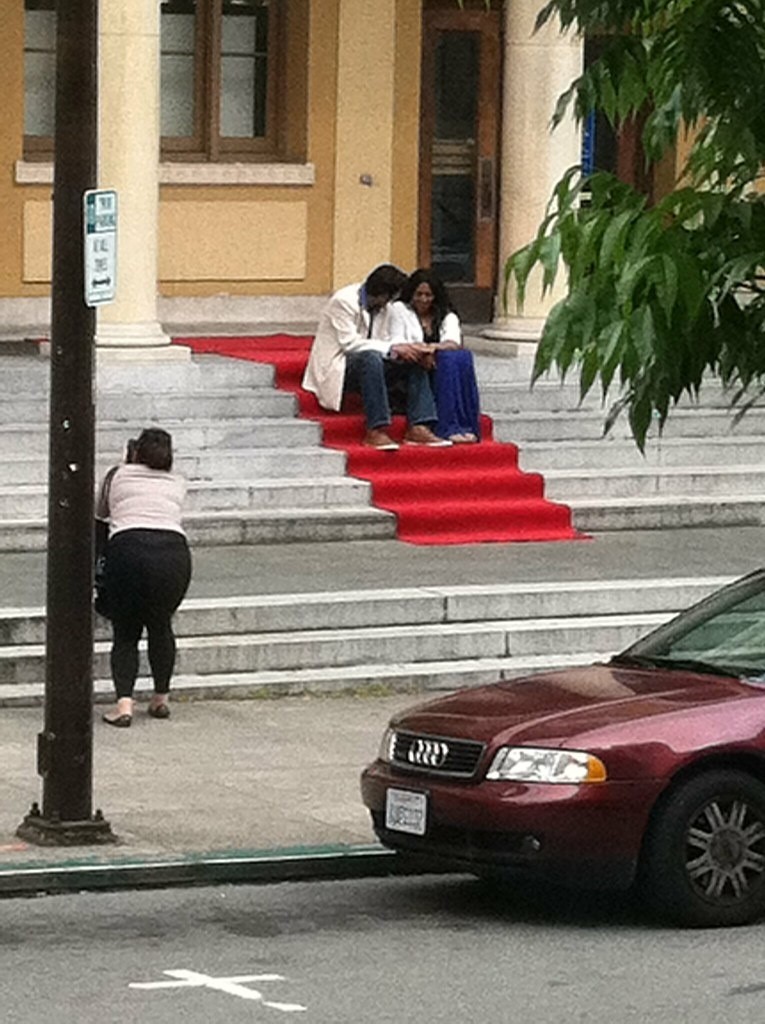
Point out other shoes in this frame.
[101,711,133,728]
[361,428,400,450]
[147,702,171,718]
[402,425,453,447]
[448,432,478,443]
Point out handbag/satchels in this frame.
[95,466,120,581]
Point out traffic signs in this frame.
[82,187,118,307]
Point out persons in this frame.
[301,263,480,450]
[94,428,192,727]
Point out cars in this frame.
[358,567,765,931]
